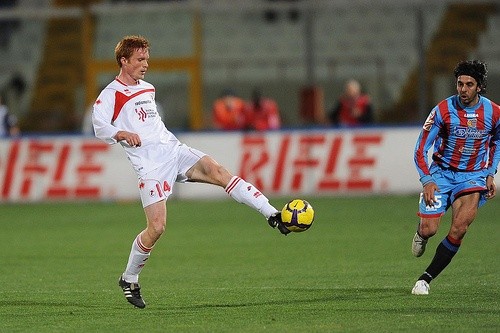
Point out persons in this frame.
[411,61,500,295]
[244,86,282,130]
[328,78,373,128]
[93,36,294,309]
[213,88,245,130]
[0,90,22,139]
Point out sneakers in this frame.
[268,213,292,235]
[118,276,146,308]
[412,279,431,295]
[412,231,427,257]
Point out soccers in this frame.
[280,198,316,234]
